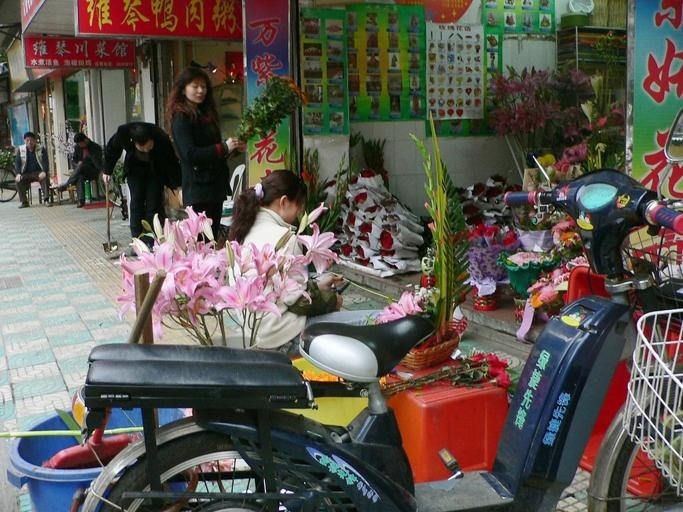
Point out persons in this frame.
[58,133,102,208]
[168,65,239,242]
[15,132,52,208]
[102,122,180,257]
[228,170,344,357]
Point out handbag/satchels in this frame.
[288,280,336,316]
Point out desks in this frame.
[214,210,234,250]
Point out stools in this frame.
[23,175,100,208]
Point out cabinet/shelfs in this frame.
[554,22,627,114]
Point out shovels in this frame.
[103,177,118,257]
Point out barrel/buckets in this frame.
[7,403,188,512]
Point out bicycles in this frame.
[0,144,19,207]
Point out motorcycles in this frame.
[65,106,683,512]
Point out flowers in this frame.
[219,70,311,165]
[99,201,343,357]
[283,63,641,401]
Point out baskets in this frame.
[620,309,682,500]
[617,228,682,323]
[400,334,460,371]
